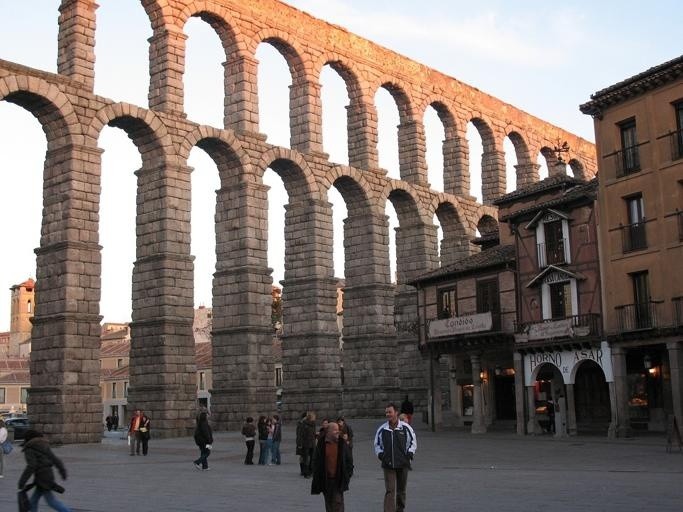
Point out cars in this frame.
[0,410,28,438]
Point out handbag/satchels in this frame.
[3,439,13,454]
[18,490,30,512]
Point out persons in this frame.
[18,430,72,512]
[398,414,409,422]
[106,409,150,456]
[241,417,256,465]
[401,395,413,424]
[193,413,213,471]
[0,420,8,478]
[258,412,354,478]
[374,403,418,512]
[311,423,352,512]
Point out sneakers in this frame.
[193,461,210,471]
